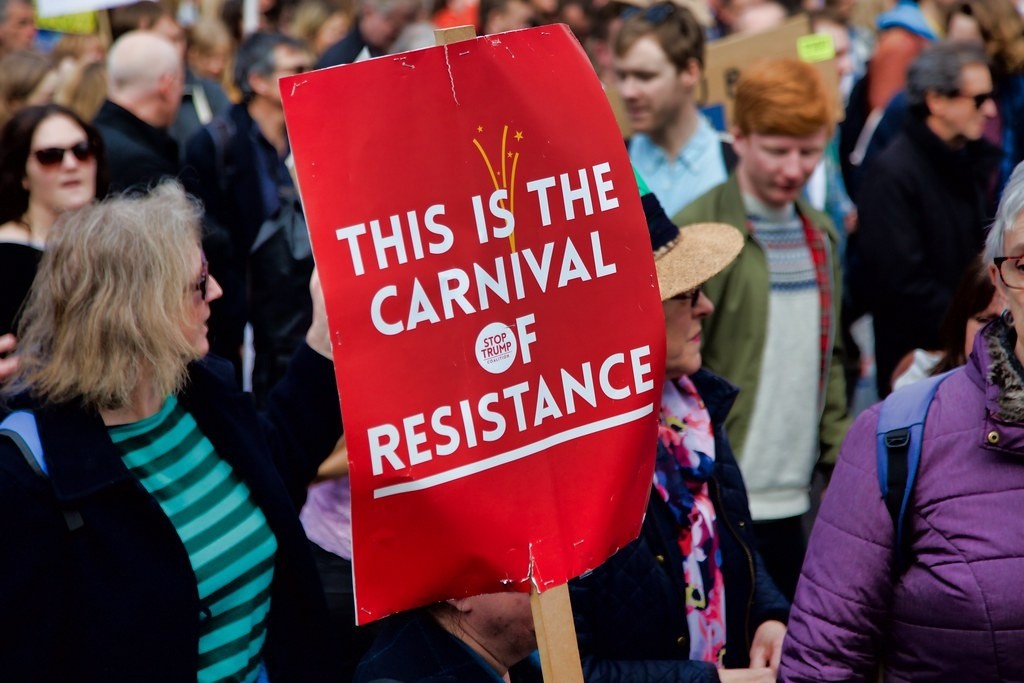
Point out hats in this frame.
[875,2,939,41]
[631,162,746,301]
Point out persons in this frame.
[0,0,1024,682]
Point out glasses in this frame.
[672,284,705,308]
[621,2,690,41]
[994,256,1024,291]
[28,142,90,164]
[939,88,995,109]
[183,250,209,300]
[268,66,316,74]
